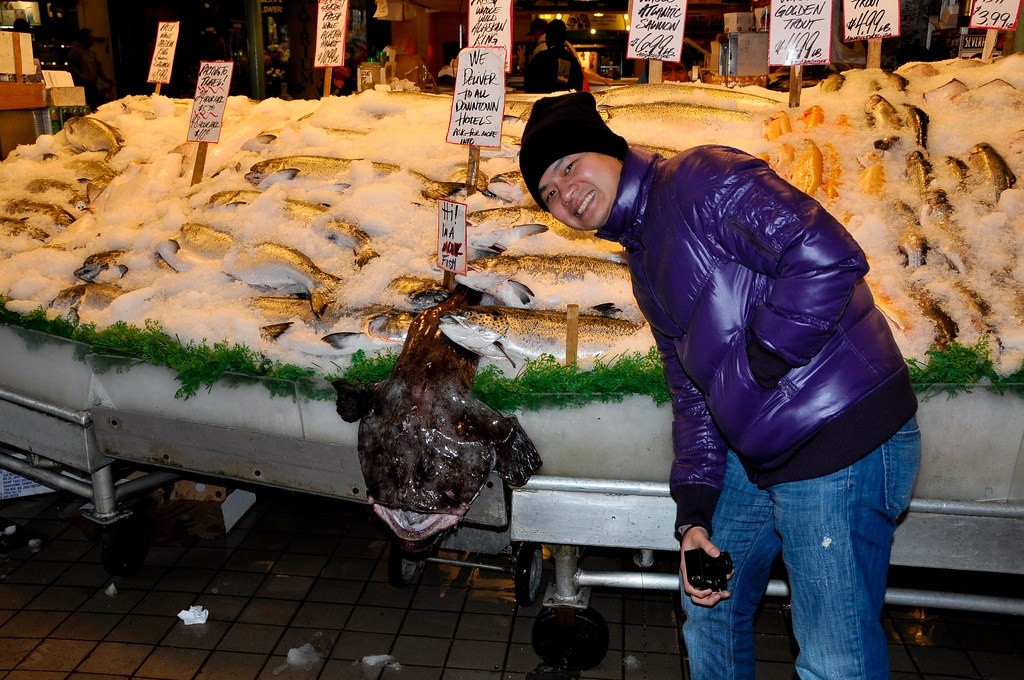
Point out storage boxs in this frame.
[723,12,754,33]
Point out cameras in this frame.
[684,548,733,593]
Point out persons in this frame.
[523,18,584,94]
[68,28,114,111]
[518,90,922,680]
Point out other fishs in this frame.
[330,281,544,543]
[0,51,1024,386]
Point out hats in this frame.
[519,92,628,211]
